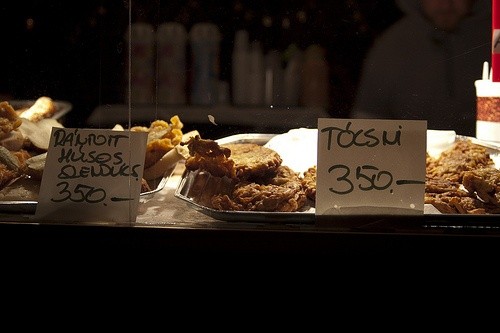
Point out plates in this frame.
[0,160,178,215]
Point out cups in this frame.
[473,79,500,143]
